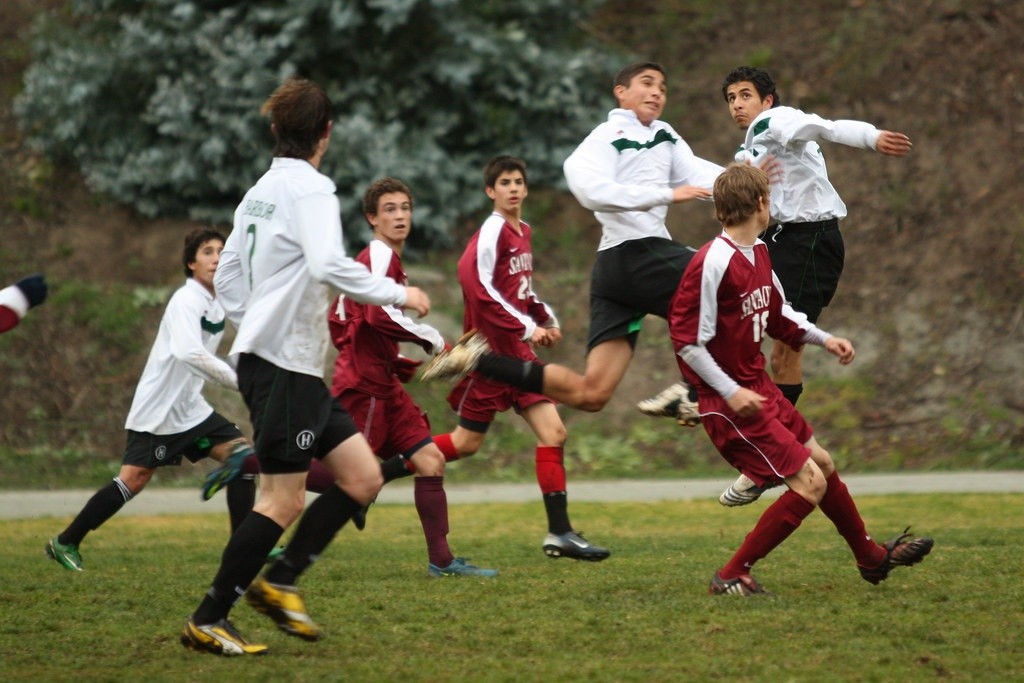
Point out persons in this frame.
[0,61,935,658]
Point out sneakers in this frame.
[353,504,372,531]
[244,573,324,641]
[637,383,689,417]
[543,530,611,561]
[419,328,490,383]
[179,615,268,658]
[718,474,764,506]
[676,392,702,427]
[200,437,255,501]
[709,569,768,597]
[857,525,934,585]
[45,536,83,573]
[428,556,498,579]
